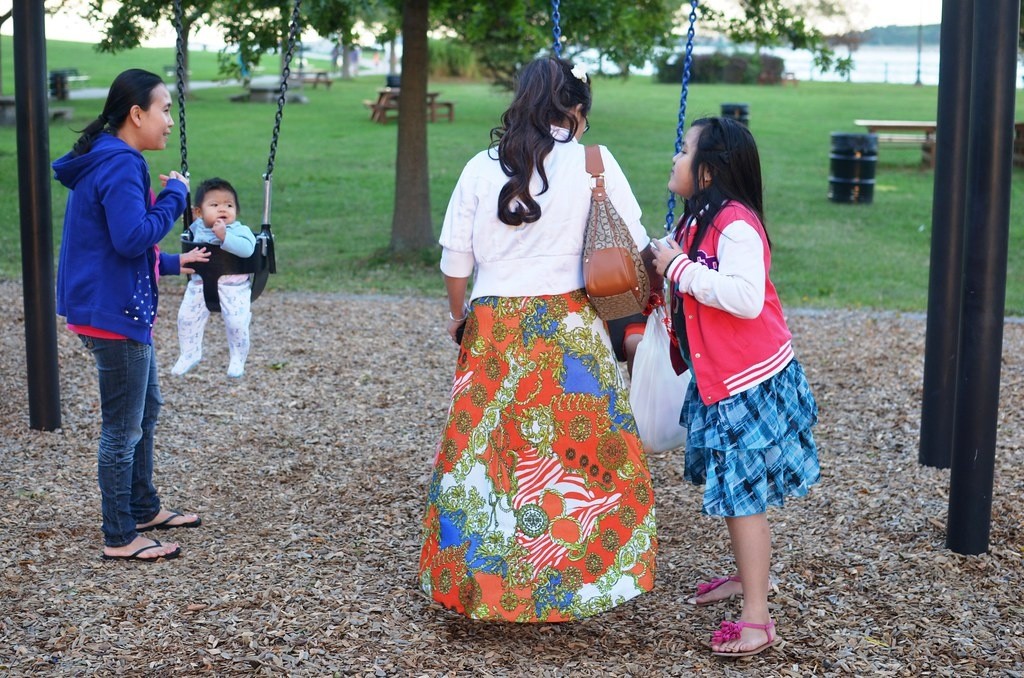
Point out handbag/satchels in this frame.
[630,306,692,454]
[582,143,649,321]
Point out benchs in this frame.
[290,77,333,91]
[854,134,937,173]
[363,102,455,125]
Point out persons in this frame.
[648,116,819,658]
[172,177,257,377]
[374,51,380,66]
[417,54,663,625]
[50,69,213,563]
[349,45,360,77]
[331,43,343,68]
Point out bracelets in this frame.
[450,304,468,322]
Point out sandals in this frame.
[713,619,781,657]
[692,576,774,607]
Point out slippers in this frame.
[103,538,181,561]
[135,510,201,532]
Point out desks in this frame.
[291,71,330,89]
[853,118,937,139]
[0,96,16,127]
[375,86,443,103]
[249,87,282,104]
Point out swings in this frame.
[172,3,303,314]
[548,0,701,364]
